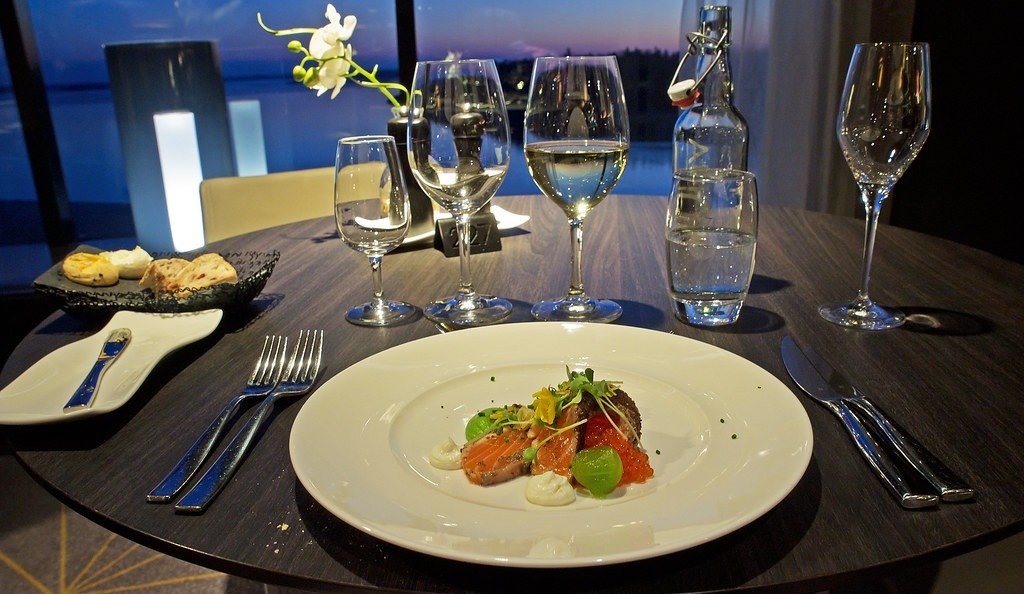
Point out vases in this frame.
[387,106,434,239]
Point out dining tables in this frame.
[0,195,1024,594]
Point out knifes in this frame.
[780,331,939,509]
[792,332,976,504]
[62,325,132,416]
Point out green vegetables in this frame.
[465,364,646,498]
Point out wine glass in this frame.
[522,54,631,322]
[410,57,513,330]
[818,40,932,331]
[332,135,418,327]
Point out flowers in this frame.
[257,4,412,111]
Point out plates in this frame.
[289,319,814,570]
[0,306,225,426]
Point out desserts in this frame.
[62,245,239,301]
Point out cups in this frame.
[662,167,760,327]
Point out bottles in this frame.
[666,4,750,175]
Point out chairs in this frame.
[199,162,396,247]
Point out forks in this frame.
[146,334,288,504]
[175,329,325,514]
